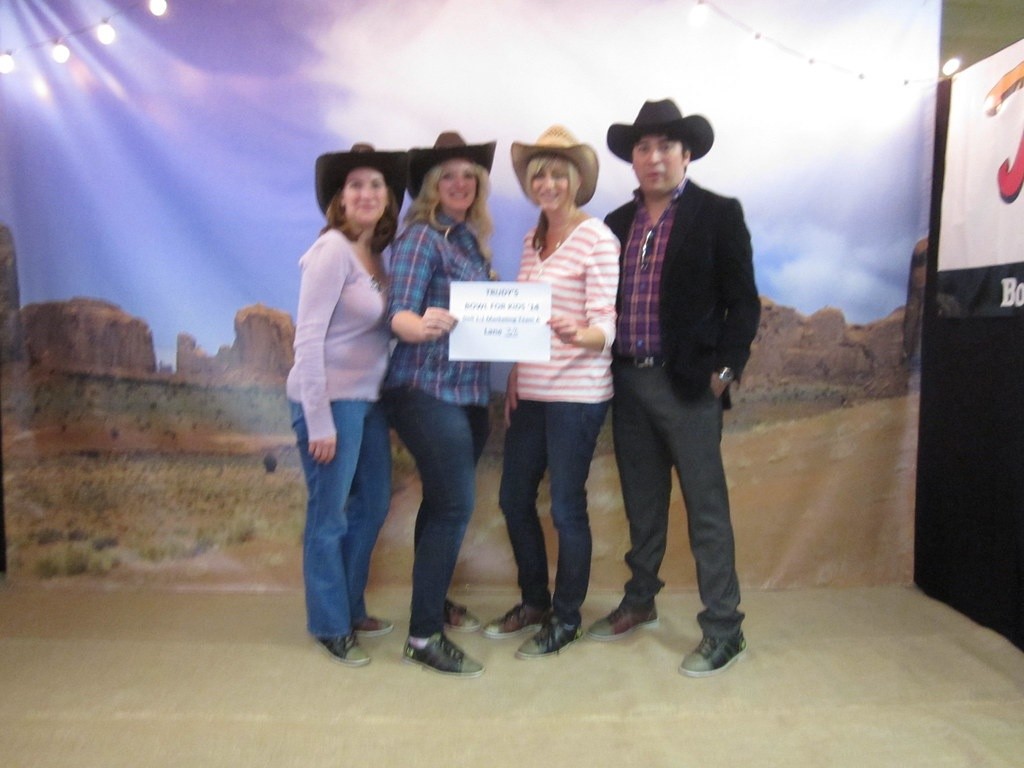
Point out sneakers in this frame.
[353,615,394,638]
[586,603,658,642]
[403,630,485,679]
[515,615,585,661]
[677,633,748,677]
[313,632,372,667]
[444,599,481,634]
[480,602,552,640]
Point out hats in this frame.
[314,143,409,218]
[606,98,714,163]
[404,132,495,199]
[510,124,600,208]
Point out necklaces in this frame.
[532,203,578,276]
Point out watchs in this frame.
[715,365,735,383]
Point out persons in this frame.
[587,97,763,679]
[380,132,503,682]
[476,121,623,663]
[284,141,406,669]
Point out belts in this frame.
[614,354,668,370]
